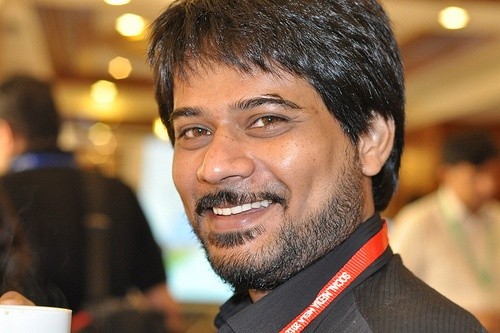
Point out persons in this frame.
[388,129,500,330]
[136,0,488,332]
[1,70,168,313]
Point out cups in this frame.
[1,305,73,333]
